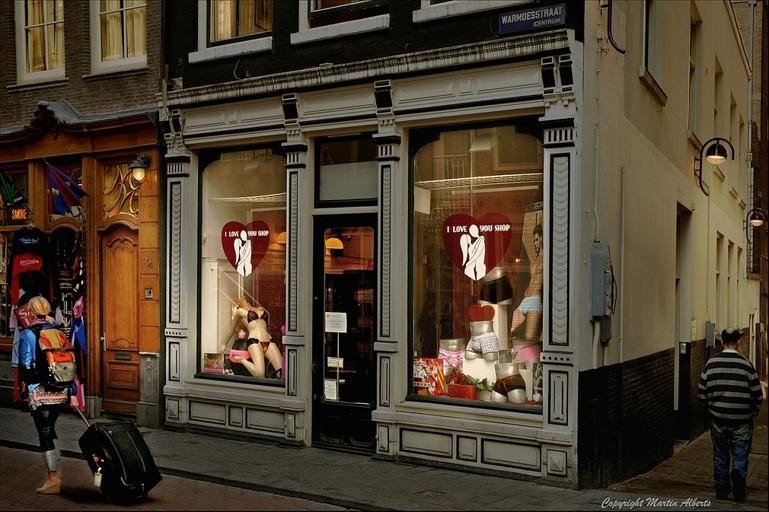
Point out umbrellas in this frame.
[46,161,93,216]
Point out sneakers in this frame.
[716,492,728,500]
[730,469,747,503]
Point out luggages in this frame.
[78,419,162,502]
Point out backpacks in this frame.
[36,327,77,393]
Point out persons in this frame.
[491,361,526,404]
[699,325,765,501]
[478,267,515,306]
[466,320,499,362]
[510,223,544,341]
[436,336,466,376]
[18,295,79,495]
[219,296,283,378]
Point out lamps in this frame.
[692,135,736,198]
[742,206,765,245]
[126,156,152,181]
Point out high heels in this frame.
[35,469,65,496]
